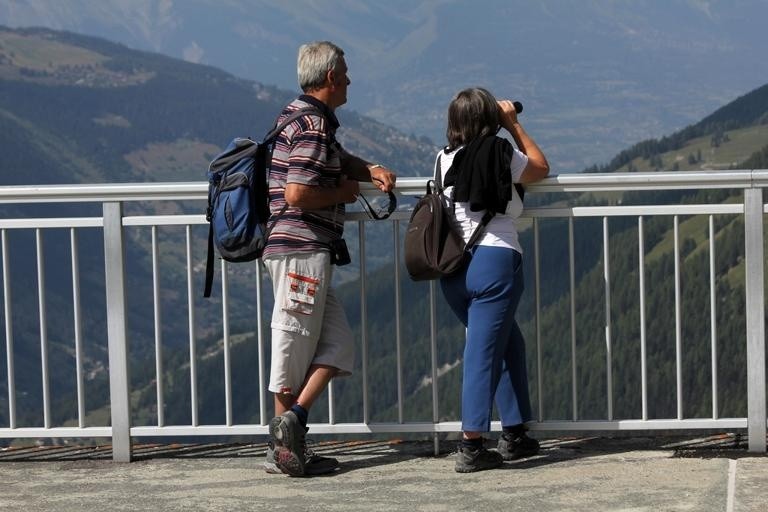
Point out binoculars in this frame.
[496,102,523,122]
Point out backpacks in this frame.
[405,154,497,281]
[205,107,327,297]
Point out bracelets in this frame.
[509,120,520,134]
[368,164,386,170]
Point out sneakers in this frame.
[498,432,539,461]
[264,410,338,477]
[454,447,503,473]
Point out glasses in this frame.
[352,176,396,221]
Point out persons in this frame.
[264,41,398,477]
[433,86,551,472]
